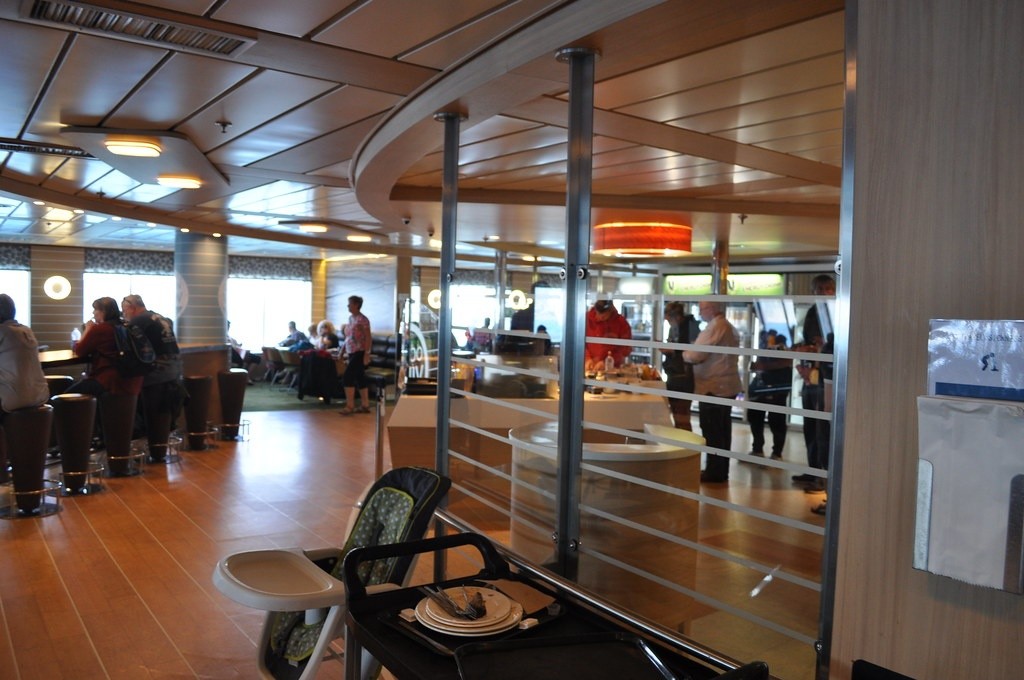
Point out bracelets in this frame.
[365,351,371,355]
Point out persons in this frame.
[747,329,793,458]
[122,295,185,439]
[60,298,145,449]
[278,320,348,397]
[789,276,834,516]
[0,293,49,484]
[403,318,550,375]
[337,295,372,414]
[228,321,255,385]
[584,300,632,369]
[654,302,701,433]
[682,293,744,483]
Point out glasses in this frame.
[124,297,132,305]
[595,303,610,313]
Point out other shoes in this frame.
[46,446,60,454]
[792,473,818,484]
[809,499,825,514]
[249,379,255,385]
[804,480,825,493]
[760,454,783,469]
[89,437,104,453]
[701,470,728,483]
[339,406,353,414]
[354,407,370,413]
[740,451,764,463]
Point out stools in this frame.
[45,376,74,395]
[141,381,183,464]
[179,375,217,452]
[7,402,62,519]
[50,393,105,496]
[217,367,251,441]
[96,390,145,480]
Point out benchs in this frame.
[336,330,395,399]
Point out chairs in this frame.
[261,347,301,391]
[215,466,452,680]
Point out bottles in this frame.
[605,350,614,371]
[71,328,81,354]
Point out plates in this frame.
[414,586,524,636]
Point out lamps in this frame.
[590,210,693,256]
[104,134,161,160]
[156,174,202,191]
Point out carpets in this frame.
[241,379,377,412]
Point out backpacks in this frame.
[99,318,157,373]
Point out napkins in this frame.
[473,579,555,614]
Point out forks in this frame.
[462,584,477,620]
[437,586,470,615]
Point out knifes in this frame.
[417,587,456,617]
[424,586,461,614]
